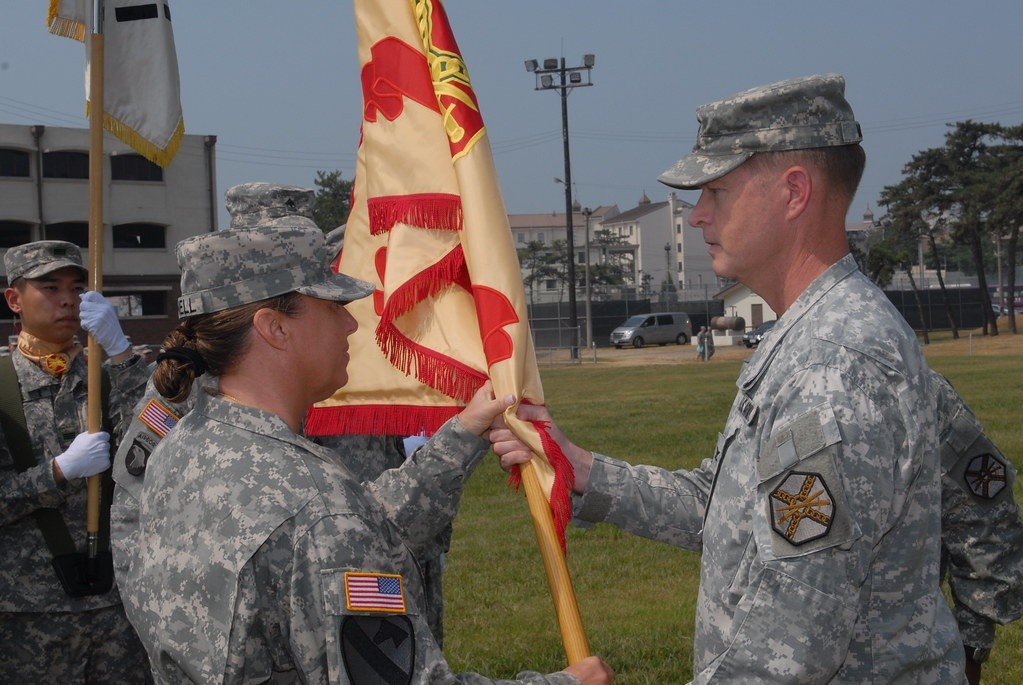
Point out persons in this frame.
[697,326,706,361]
[126,335,152,365]
[108,183,445,653]
[9,335,19,352]
[125,214,613,685]
[705,327,714,361]
[0,240,153,685]
[488,73,1023,685]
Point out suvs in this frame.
[609,311,692,349]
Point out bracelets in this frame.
[965,645,989,664]
[112,352,139,367]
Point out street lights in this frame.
[663,242,673,312]
[523,54,598,358]
[579,207,593,350]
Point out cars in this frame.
[742,320,778,348]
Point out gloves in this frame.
[54,430,112,481]
[79,291,129,356]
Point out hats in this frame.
[3,240,89,288]
[657,74,863,190]
[174,215,376,319]
[225,182,315,227]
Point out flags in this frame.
[46,0,185,169]
[303,0,576,561]
